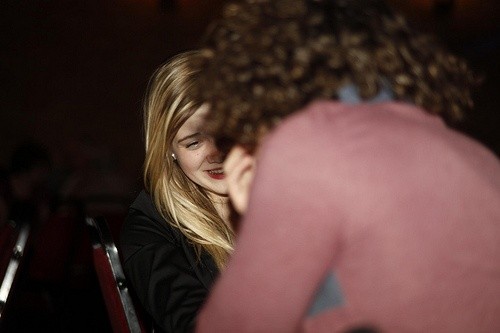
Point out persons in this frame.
[118,50,256,333]
[195,0,500,333]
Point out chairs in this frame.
[94,239,144,333]
